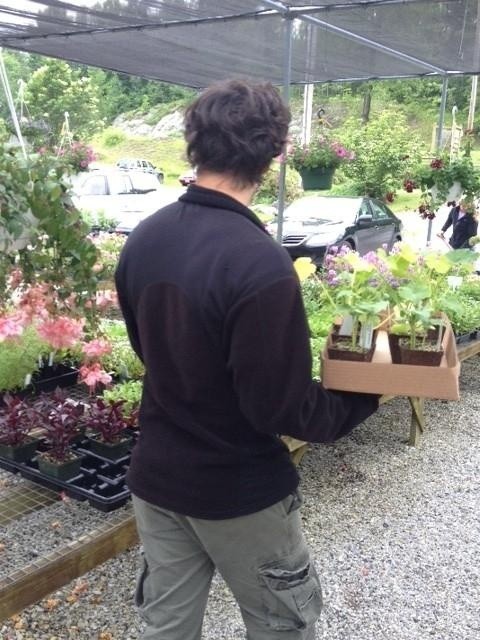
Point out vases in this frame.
[431,176,464,202]
[1,204,35,254]
[29,363,80,392]
[299,167,334,190]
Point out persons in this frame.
[440,196,477,249]
[112,75,382,639]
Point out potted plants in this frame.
[0,380,138,480]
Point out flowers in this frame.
[0,271,122,394]
[402,154,480,225]
[280,130,349,169]
[0,138,112,295]
[318,242,456,365]
